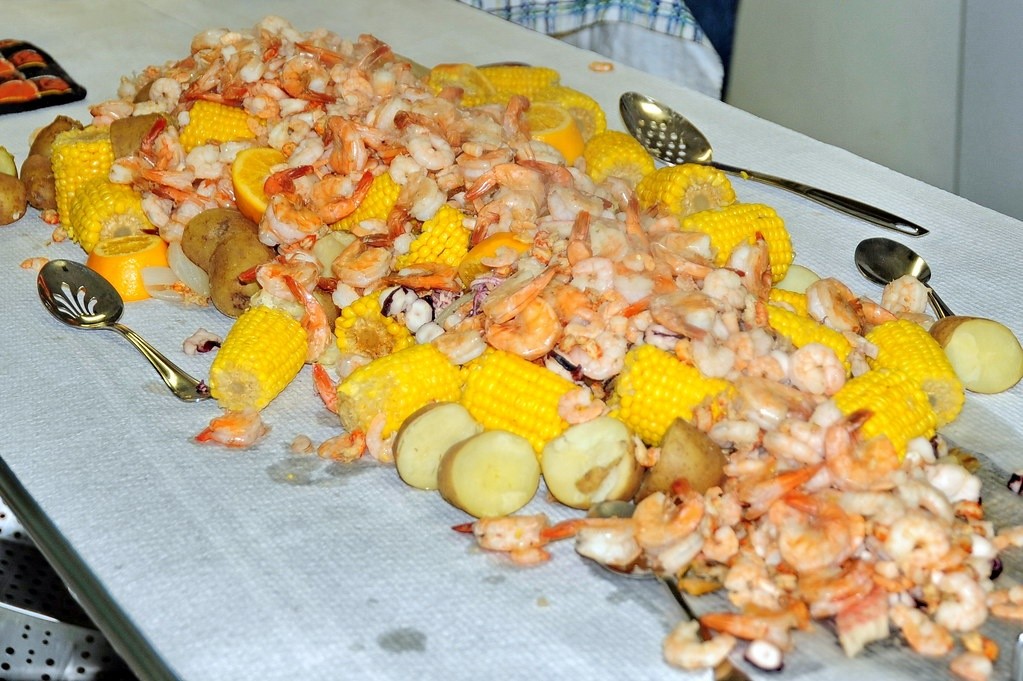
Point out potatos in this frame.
[927,313,1023,394]
[392,400,726,521]
[0,114,82,225]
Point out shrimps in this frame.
[86,14,1023,681]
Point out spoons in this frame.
[850,235,952,321]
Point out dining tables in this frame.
[0,0,1023,681]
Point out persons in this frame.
[687,1,739,104]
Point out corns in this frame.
[435,57,963,453]
[48,98,587,467]
